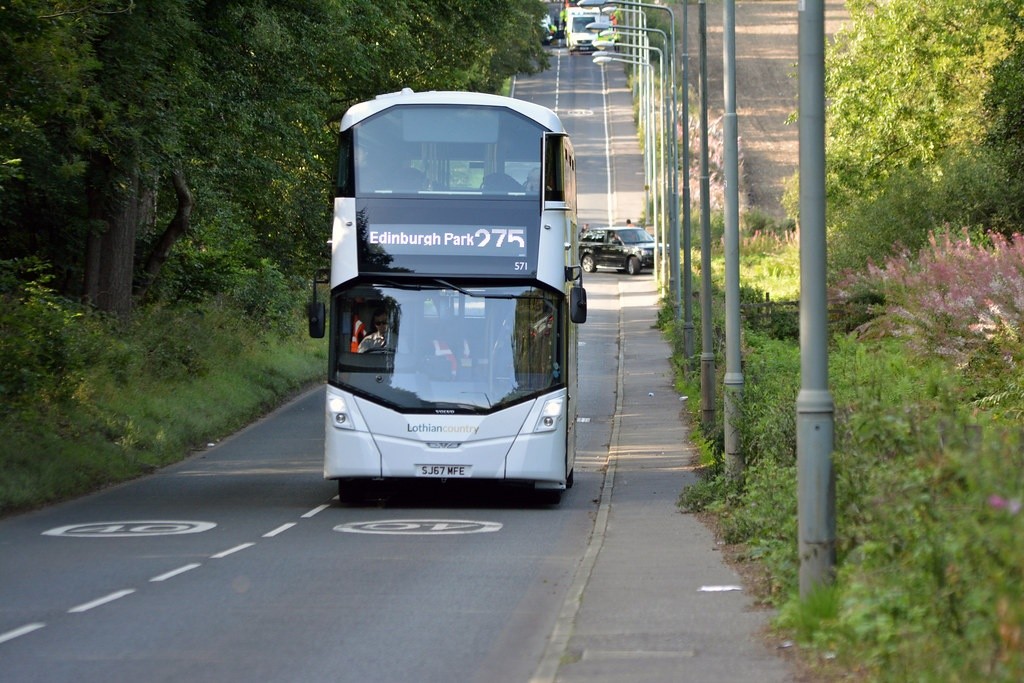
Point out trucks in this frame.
[567,6,600,56]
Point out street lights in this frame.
[577,0,682,323]
[602,6,646,135]
[592,41,667,299]
[600,30,648,200]
[584,21,674,291]
[592,56,659,279]
[591,50,651,217]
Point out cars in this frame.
[540,15,554,45]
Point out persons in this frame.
[358,308,402,353]
[549,10,566,33]
[626,219,634,227]
[582,224,589,232]
[526,167,543,192]
[610,9,623,52]
[609,232,637,243]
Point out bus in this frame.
[308,88,587,504]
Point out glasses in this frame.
[376,320,389,326]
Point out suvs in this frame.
[579,227,671,275]
